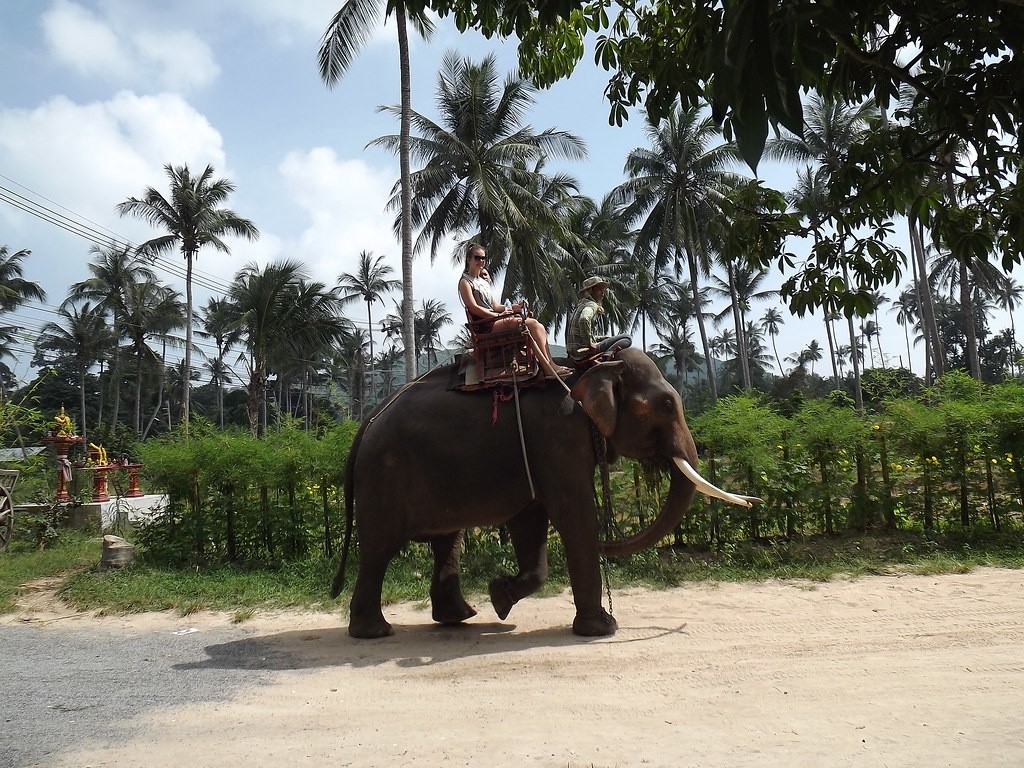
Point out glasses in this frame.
[469,255,484,262]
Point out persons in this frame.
[458,241,574,379]
[567,276,632,364]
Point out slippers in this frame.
[545,365,573,379]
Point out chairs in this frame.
[464,306,533,384]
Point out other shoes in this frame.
[592,356,611,365]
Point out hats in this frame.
[578,276,609,294]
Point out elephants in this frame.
[327,346,768,639]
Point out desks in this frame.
[43,436,84,502]
[76,464,142,502]
